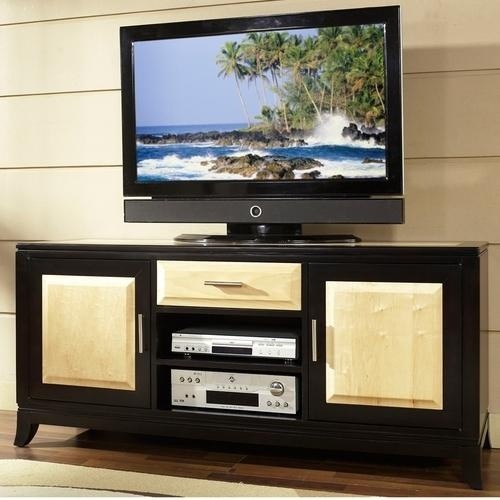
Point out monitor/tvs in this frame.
[121,5,405,245]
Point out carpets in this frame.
[1,457,369,497]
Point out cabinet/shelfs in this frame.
[13,242,491,491]
